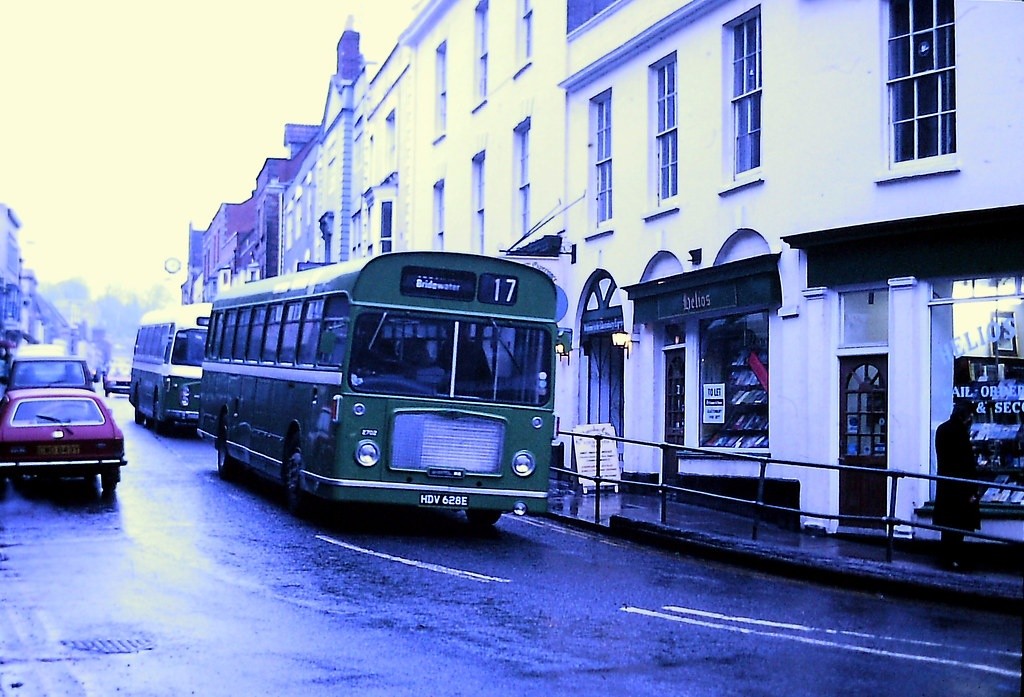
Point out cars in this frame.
[0,388,128,495]
[102,357,132,397]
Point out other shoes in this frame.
[936,557,966,574]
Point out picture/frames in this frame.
[988,310,1019,358]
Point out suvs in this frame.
[0,357,102,392]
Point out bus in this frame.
[197,251,573,525]
[129,303,213,436]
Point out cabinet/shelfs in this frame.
[711,344,767,447]
[953,355,1023,511]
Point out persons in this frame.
[931,400,996,570]
[58,365,81,383]
[437,322,492,382]
[19,366,39,384]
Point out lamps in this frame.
[611,323,632,360]
[555,343,570,366]
